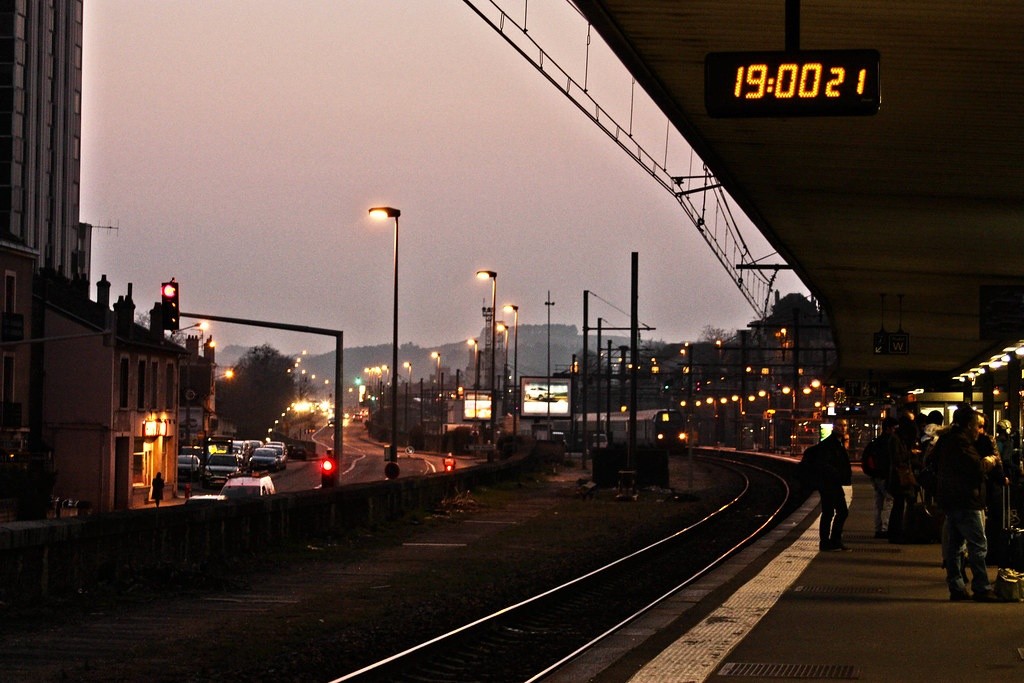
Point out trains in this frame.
[519,408,687,453]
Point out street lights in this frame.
[496,323,509,416]
[503,304,519,452]
[468,338,480,390]
[403,361,412,394]
[476,270,498,462]
[432,352,441,393]
[367,207,402,461]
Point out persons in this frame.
[863,409,1024,602]
[818,416,852,551]
[151,472,164,507]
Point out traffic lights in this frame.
[320,458,336,474]
[160,281,180,331]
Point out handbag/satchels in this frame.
[994,568,1024,601]
[896,465,918,487]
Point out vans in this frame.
[219,475,276,498]
[205,435,235,454]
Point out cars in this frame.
[233,439,308,475]
[184,495,229,505]
[526,387,556,400]
[352,409,368,422]
[178,445,206,481]
[327,416,336,426]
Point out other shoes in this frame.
[972,588,999,602]
[875,529,889,538]
[950,588,974,601]
[819,541,853,553]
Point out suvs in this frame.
[201,452,241,490]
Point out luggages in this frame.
[998,484,1024,573]
